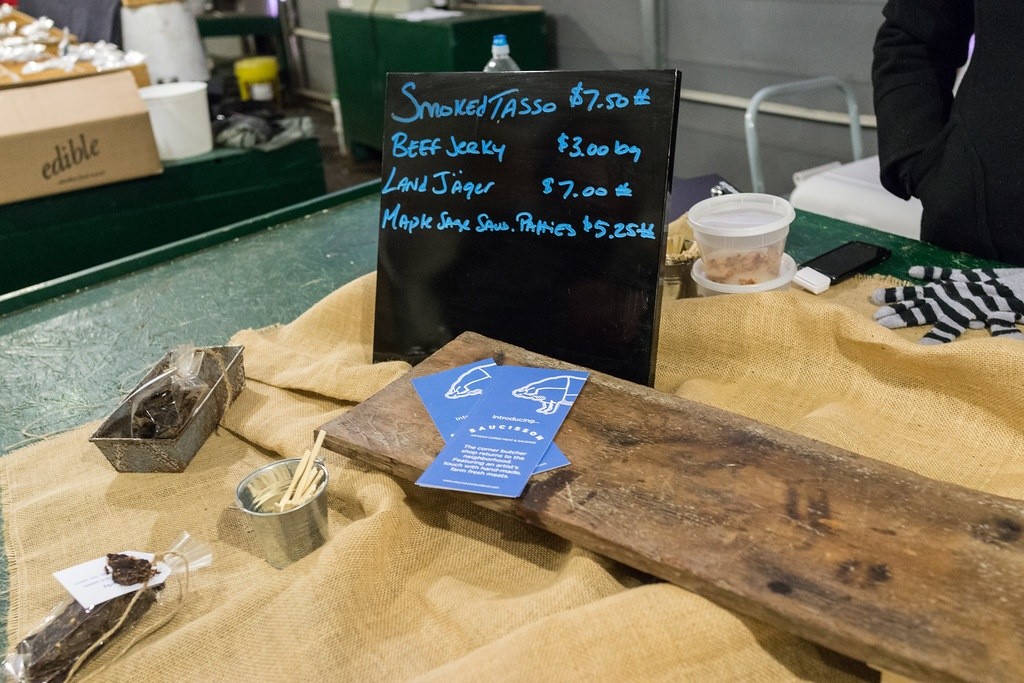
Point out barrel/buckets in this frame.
[232,55,282,113]
[140,81,213,161]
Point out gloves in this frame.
[872,265,1024,344]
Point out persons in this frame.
[870,0,1024,266]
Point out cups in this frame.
[235,458,329,568]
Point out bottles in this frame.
[482,34,521,72]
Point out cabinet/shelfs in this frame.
[328,5,549,164]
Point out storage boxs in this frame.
[0,12,165,205]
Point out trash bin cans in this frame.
[235,56,282,106]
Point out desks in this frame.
[0,136,1023,683]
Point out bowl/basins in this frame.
[691,191,799,303]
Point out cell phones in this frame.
[798,241,892,285]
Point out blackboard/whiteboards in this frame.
[374,68,683,389]
